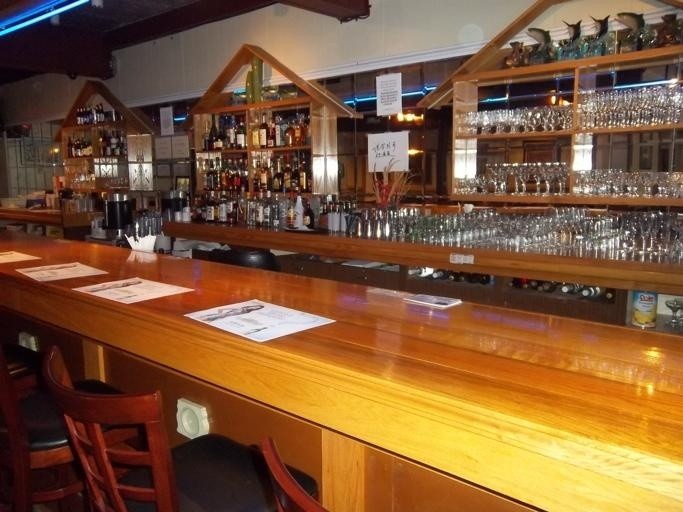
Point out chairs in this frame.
[251,435,328,511]
[42,344,180,512]
[0,350,147,512]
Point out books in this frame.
[403,294,462,308]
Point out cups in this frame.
[174,207,191,224]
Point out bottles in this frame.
[357,207,418,239]
[72,139,87,158]
[408,266,616,305]
[200,112,356,235]
[76,103,104,124]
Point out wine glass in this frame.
[456,161,572,196]
[573,166,683,200]
[454,207,682,263]
[577,84,682,131]
[454,83,572,136]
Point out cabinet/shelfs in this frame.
[163,222,683,336]
[52,81,157,192]
[416,0,682,210]
[180,44,363,228]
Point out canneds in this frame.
[631,292,658,330]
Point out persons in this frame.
[200,305,266,321]
[86,281,142,292]
